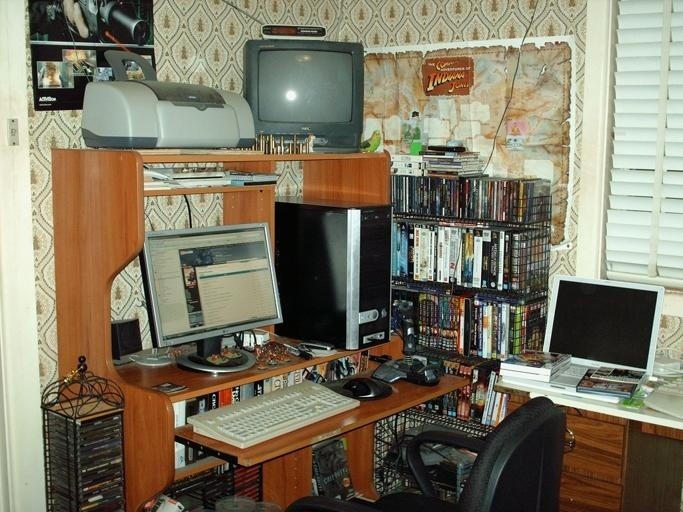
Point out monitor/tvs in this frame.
[138,222,284,373]
[243,40,365,153]
[542,275,665,376]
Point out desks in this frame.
[493,352,683,512]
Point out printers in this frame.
[81,50,255,149]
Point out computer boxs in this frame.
[274,195,394,350]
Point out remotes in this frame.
[428,145,467,152]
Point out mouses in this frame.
[342,375,392,399]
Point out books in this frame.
[313,439,356,501]
[173,353,367,471]
[497,295,549,349]
[391,174,552,225]
[409,356,507,427]
[393,288,496,361]
[499,361,572,384]
[592,366,646,385]
[577,369,637,399]
[392,219,550,292]
[500,350,571,383]
[390,150,490,178]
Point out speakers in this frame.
[111,318,143,365]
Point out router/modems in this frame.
[236,329,270,347]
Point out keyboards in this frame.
[187,380,360,449]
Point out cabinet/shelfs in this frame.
[52,147,471,512]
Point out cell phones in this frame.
[300,339,335,351]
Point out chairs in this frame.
[285,396,566,512]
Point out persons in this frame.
[38,63,61,88]
[62,0,91,39]
